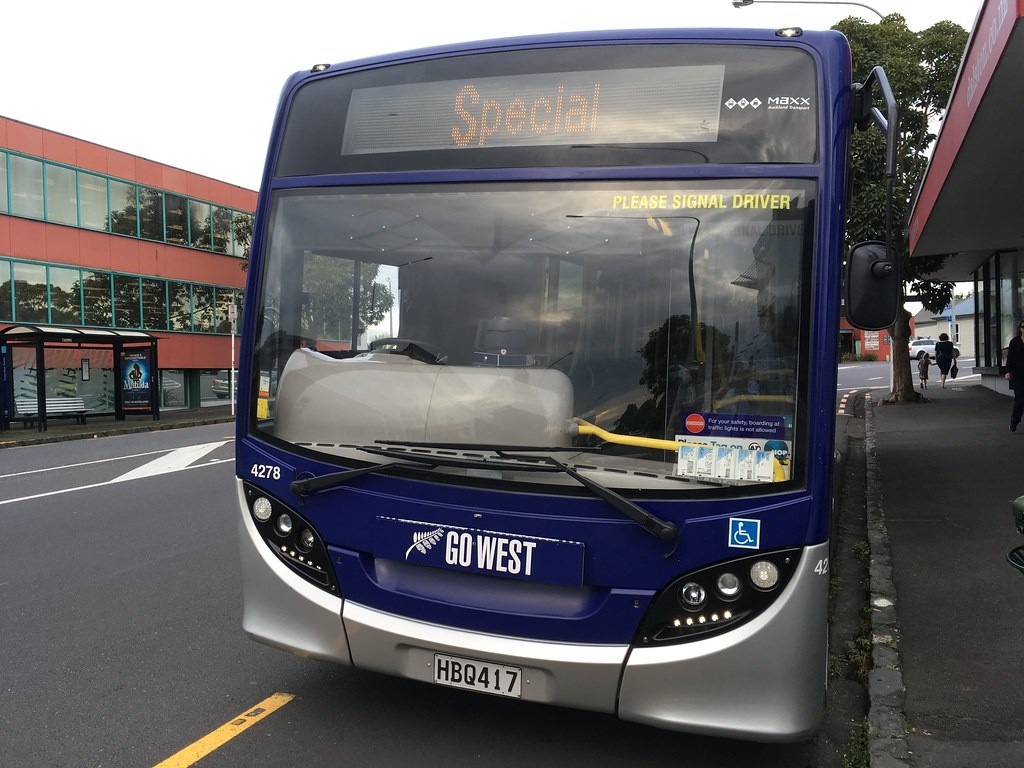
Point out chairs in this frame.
[474,308,540,358]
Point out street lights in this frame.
[729,0,893,29]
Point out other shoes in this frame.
[1009,419,1018,432]
[920,383,923,389]
[941,383,944,389]
[923,386,927,390]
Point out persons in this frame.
[935,333,956,387]
[1005,320,1024,432]
[918,353,931,390]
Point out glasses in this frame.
[1021,324,1024,330]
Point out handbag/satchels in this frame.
[951,363,959,379]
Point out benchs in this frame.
[14,398,95,429]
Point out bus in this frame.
[224,23,904,739]
[257,369,278,398]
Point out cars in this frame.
[908,339,961,360]
[211,369,239,400]
